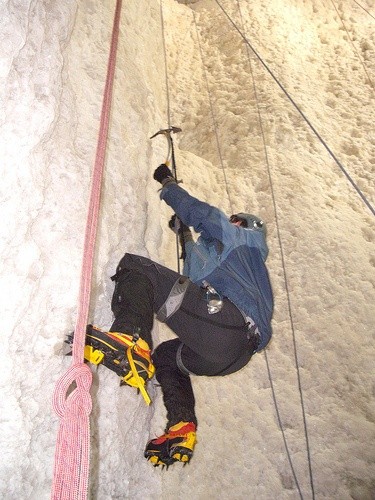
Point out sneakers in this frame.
[142,421,198,471]
[64,325,155,392]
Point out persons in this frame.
[66,164,276,468]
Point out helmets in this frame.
[236,212,266,234]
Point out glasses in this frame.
[229,216,245,223]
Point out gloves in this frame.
[169,213,191,236]
[153,163,173,184]
